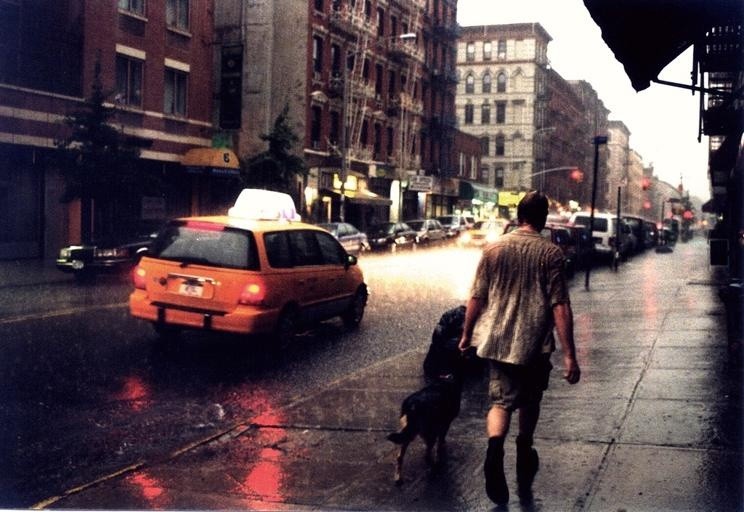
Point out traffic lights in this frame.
[642,177,649,191]
[577,171,584,185]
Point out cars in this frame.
[54,214,182,282]
[123,185,370,348]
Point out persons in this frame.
[457,189,581,507]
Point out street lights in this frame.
[338,31,419,223]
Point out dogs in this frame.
[384,376,465,485]
